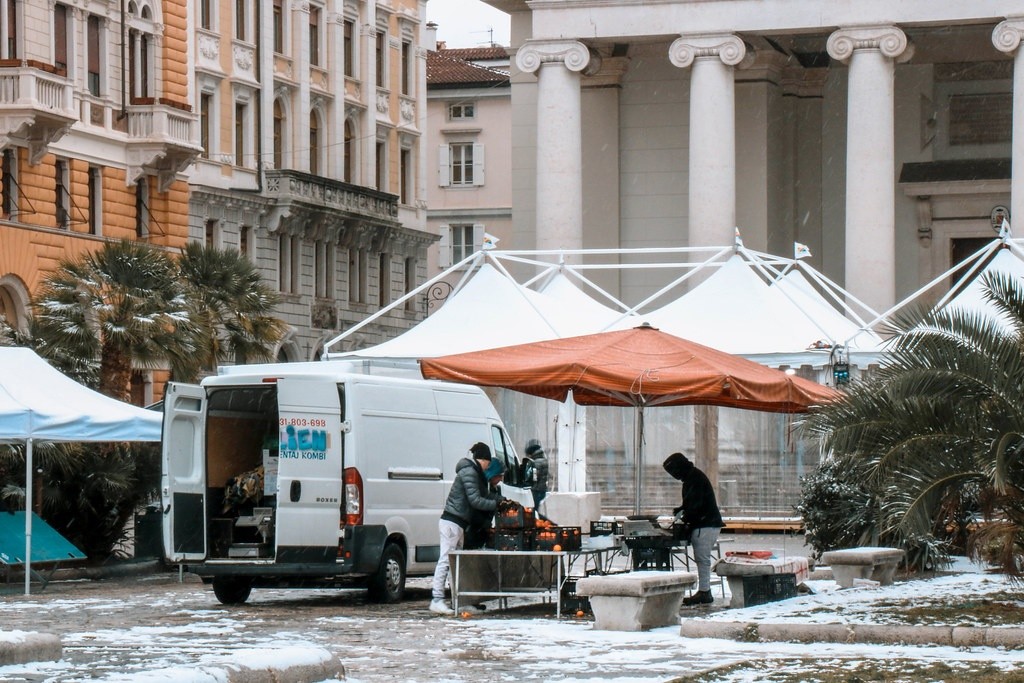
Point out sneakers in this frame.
[429,599,455,616]
[458,605,484,615]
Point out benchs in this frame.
[713,555,817,609]
[821,546,907,588]
[576,569,698,635]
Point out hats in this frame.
[470,442,491,461]
[484,457,505,480]
[526,444,540,455]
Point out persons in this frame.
[465,458,508,612]
[520,445,548,509]
[662,452,724,605]
[428,442,520,615]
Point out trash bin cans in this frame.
[134,502,161,558]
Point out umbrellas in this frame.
[1,346,163,593]
[416,318,853,520]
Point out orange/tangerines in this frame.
[486,507,579,551]
[462,612,470,617]
[576,611,584,616]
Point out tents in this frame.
[322,215,1024,522]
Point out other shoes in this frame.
[474,604,486,610]
[683,590,713,605]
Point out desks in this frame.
[446,545,621,620]
[601,538,733,596]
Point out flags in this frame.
[998,216,1013,241]
[481,232,500,250]
[735,225,744,247]
[794,240,812,261]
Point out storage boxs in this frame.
[487,502,582,553]
[742,573,797,608]
[591,519,623,539]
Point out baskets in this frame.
[494,506,582,550]
[590,520,624,537]
